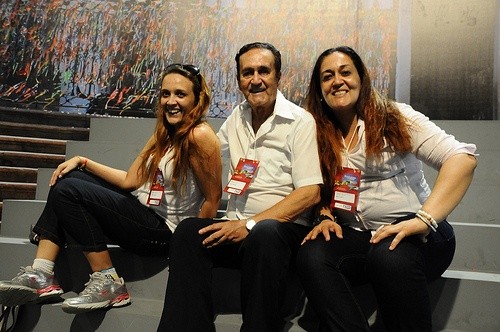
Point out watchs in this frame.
[312,214,332,227]
[245,218,256,230]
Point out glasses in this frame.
[164,64,201,88]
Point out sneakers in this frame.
[61,271,131,314]
[0,266,64,306]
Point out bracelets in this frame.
[416,213,436,232]
[418,210,438,228]
[80,161,86,169]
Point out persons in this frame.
[0,63,222,313]
[294,46,479,332]
[157,42,324,332]
